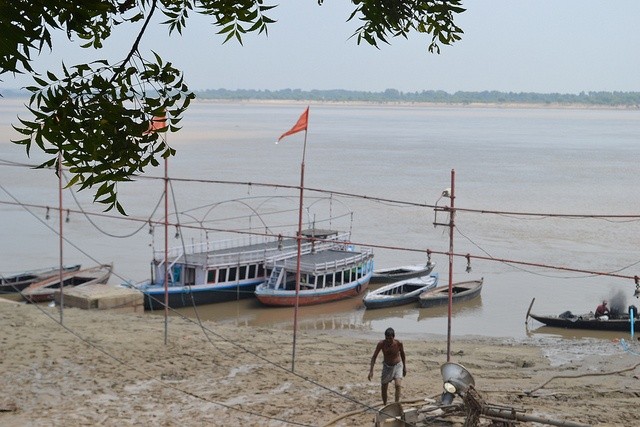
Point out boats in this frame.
[21,264,113,302]
[528,312,639,332]
[370,262,435,284]
[418,280,482,307]
[364,273,438,308]
[0,264,81,292]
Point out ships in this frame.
[255,247,373,307]
[119,196,354,309]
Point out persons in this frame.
[368,327,406,405]
[595,300,610,315]
